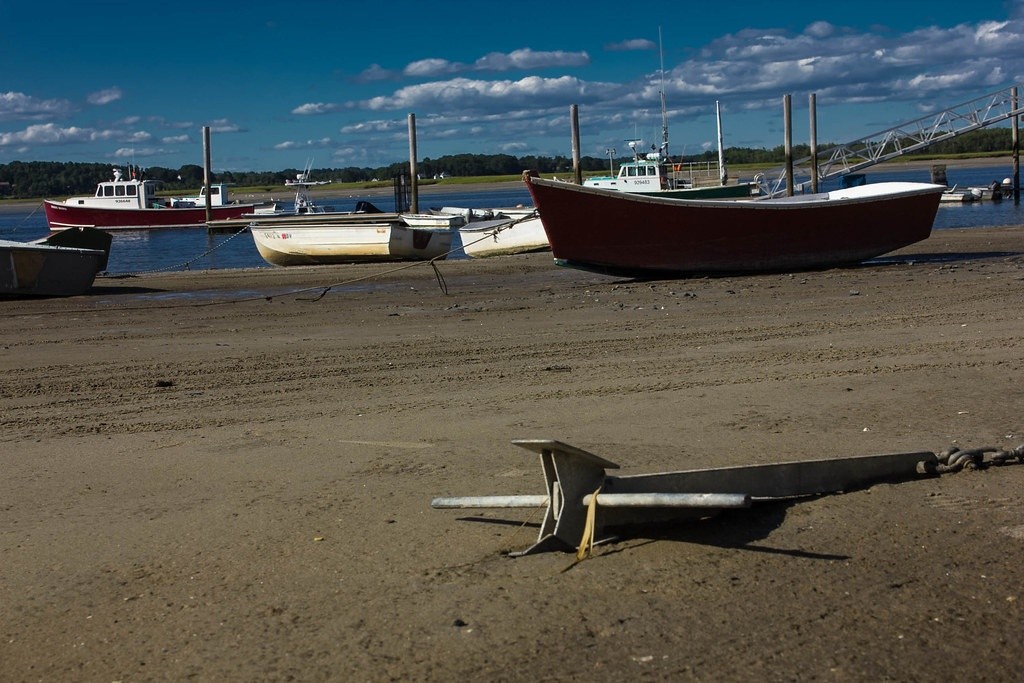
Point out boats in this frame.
[240,187,366,219]
[522,168,948,279]
[966,178,1014,202]
[248,222,455,263]
[940,189,975,204]
[41,165,265,231]
[400,207,550,259]
[582,26,695,193]
[0,227,113,300]
[284,156,332,188]
